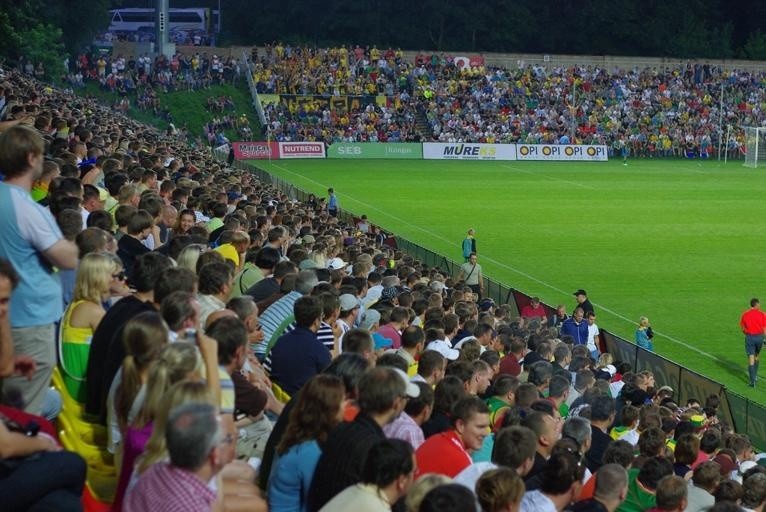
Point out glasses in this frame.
[112,271,126,282]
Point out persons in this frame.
[488,57,765,159]
[448,288,766,512]
[1,29,497,512]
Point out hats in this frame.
[572,290,587,295]
[712,455,739,475]
[298,235,460,396]
